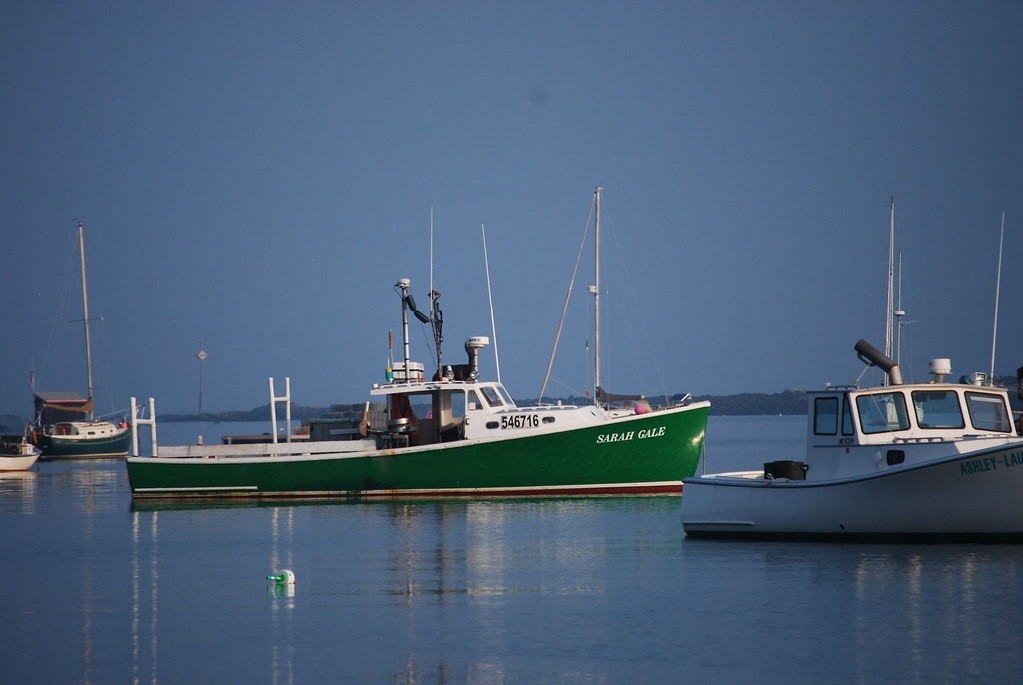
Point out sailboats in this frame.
[32,220,147,459]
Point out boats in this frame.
[681,199,1023,547]
[1,471,37,484]
[0,442,44,473]
[123,184,714,499]
